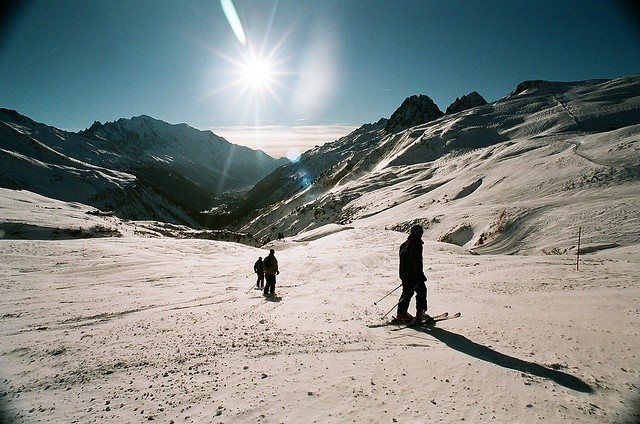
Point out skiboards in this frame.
[365,312,461,328]
[246,281,265,290]
[246,291,282,299]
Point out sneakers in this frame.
[417,309,430,320]
[397,312,414,320]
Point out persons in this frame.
[254,257,264,288]
[262,249,279,294]
[277,232,284,241]
[397,224,434,322]
